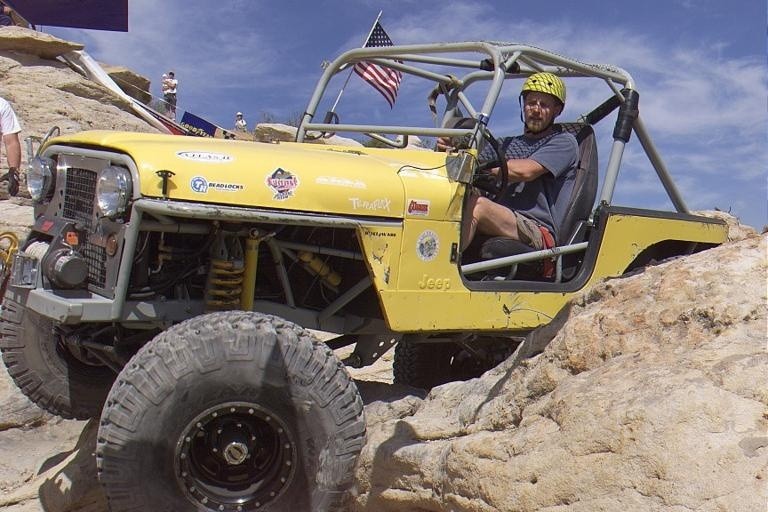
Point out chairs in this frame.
[475,123,600,280]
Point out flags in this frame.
[352,20,408,111]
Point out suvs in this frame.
[2,40,732,511]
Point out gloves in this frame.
[0,167,19,197]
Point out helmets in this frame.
[521,72,566,107]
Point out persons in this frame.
[163,70,179,121]
[0,95,23,197]
[232,111,248,133]
[437,71,581,271]
